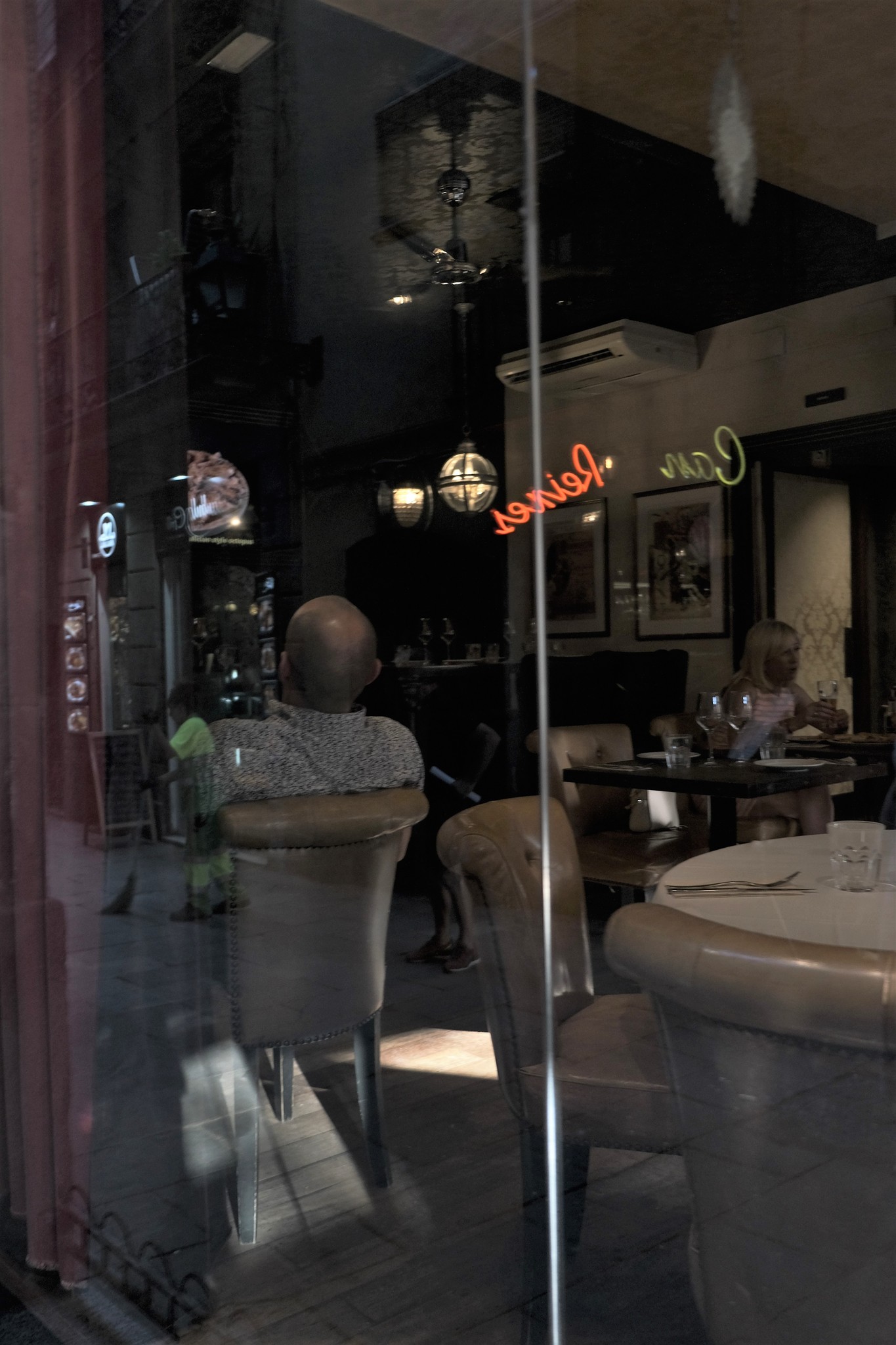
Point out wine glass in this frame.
[440,618,455,665]
[527,619,536,654]
[418,618,432,660]
[503,619,519,664]
[726,691,753,766]
[695,692,724,767]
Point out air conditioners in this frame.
[497,319,700,396]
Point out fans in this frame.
[380,122,558,311]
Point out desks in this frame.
[651,829,896,957]
[788,726,896,761]
[563,748,891,850]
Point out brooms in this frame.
[97,757,152,916]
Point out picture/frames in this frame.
[527,495,611,641]
[632,477,731,638]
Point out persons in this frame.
[122,682,248,925]
[690,617,849,843]
[176,596,425,832]
[399,639,501,975]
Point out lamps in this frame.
[435,166,503,514]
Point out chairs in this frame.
[654,713,792,845]
[602,900,896,1345]
[198,791,428,1246]
[523,724,709,913]
[437,792,687,1324]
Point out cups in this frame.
[826,820,886,892]
[757,727,787,760]
[485,644,499,664]
[466,644,481,660]
[817,679,838,710]
[662,734,692,767]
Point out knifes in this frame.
[667,886,816,893]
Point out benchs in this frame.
[510,650,689,794]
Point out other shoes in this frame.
[212,896,251,915]
[169,902,211,923]
[403,935,456,965]
[445,938,482,974]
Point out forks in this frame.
[665,870,801,887]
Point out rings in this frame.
[815,711,818,717]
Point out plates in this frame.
[753,759,826,768]
[636,751,701,759]
[392,660,431,667]
[827,738,896,745]
[785,738,827,743]
[442,659,483,664]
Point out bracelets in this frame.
[785,717,792,734]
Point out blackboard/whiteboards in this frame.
[86,728,156,830]
